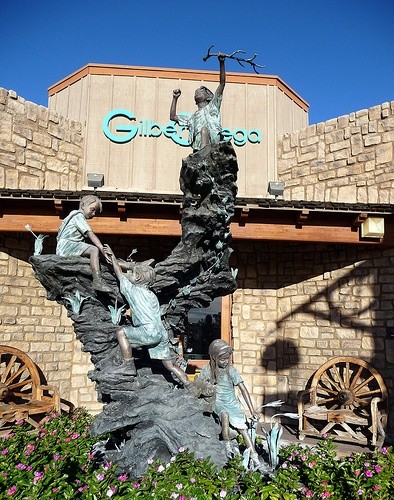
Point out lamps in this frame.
[87,173,105,190]
[361,218,384,239]
[267,181,285,201]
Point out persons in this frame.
[57,195,114,292]
[196,339,268,474]
[103,243,202,398]
[170,54,226,153]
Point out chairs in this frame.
[0,345,61,431]
[297,356,391,451]
